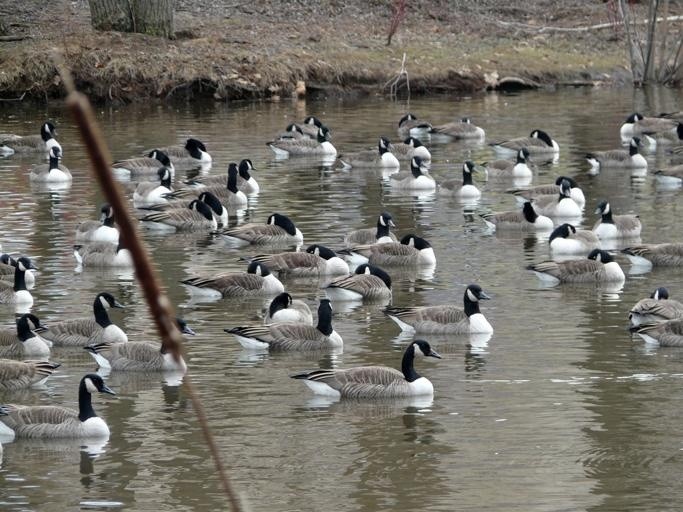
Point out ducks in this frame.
[1,112,682,446]
